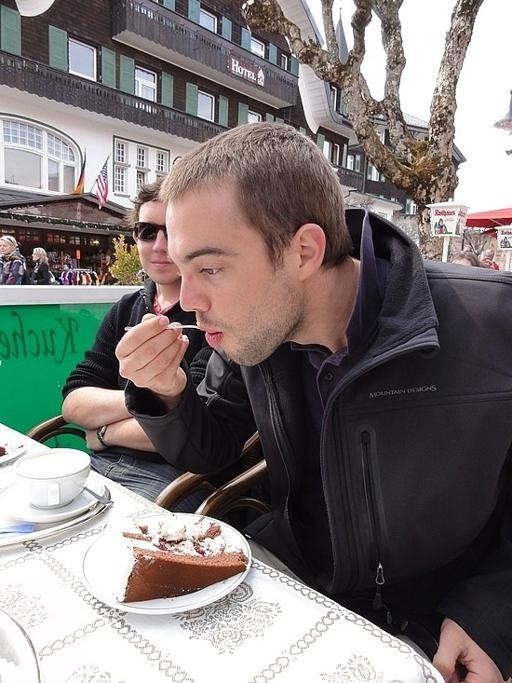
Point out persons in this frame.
[98,256,114,285]
[477,247,499,271]
[61,180,214,514]
[450,251,484,267]
[116,121,511,682]
[0,235,27,285]
[30,247,56,285]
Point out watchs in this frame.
[96,424,110,449]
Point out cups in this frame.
[14,447,93,509]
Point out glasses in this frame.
[134,221,168,240]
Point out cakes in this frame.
[115,522,248,602]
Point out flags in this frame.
[95,160,108,210]
[69,151,86,195]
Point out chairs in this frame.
[194,457,268,518]
[27,413,262,509]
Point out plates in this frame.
[0,440,27,468]
[80,509,252,615]
[2,482,108,523]
[1,488,110,547]
